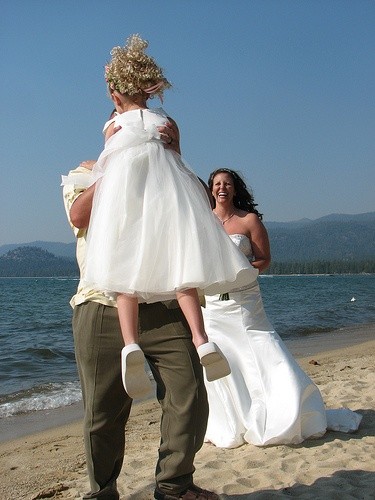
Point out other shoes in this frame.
[154,483,220,500]
[197,341,232,382]
[82,492,119,500]
[121,343,152,399]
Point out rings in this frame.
[167,139,172,144]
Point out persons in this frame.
[60,33,259,401]
[64,109,220,500]
[192,169,271,450]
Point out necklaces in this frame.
[213,208,238,225]
